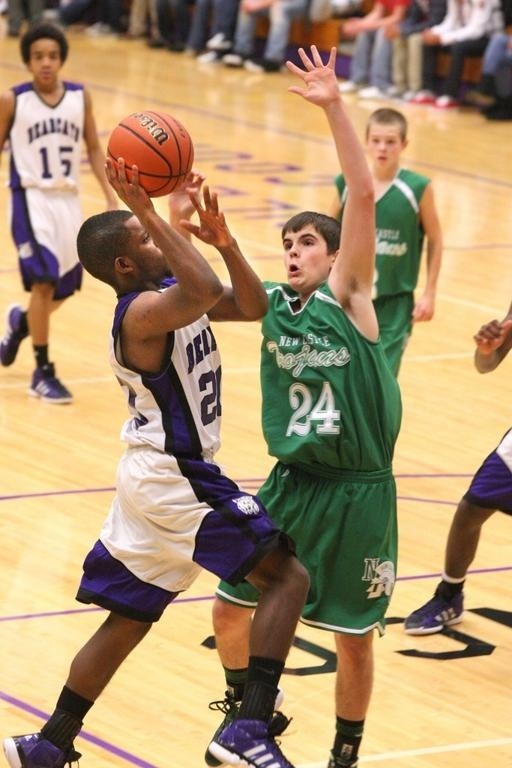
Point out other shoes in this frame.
[6,28,20,38]
[482,100,509,119]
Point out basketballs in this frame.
[108,111,193,198]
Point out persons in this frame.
[165,43,401,768]
[309,1,511,122]
[403,301,512,633]
[325,108,443,380]
[2,155,313,767]
[5,0,309,72]
[1,23,119,404]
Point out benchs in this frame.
[183,0,512,109]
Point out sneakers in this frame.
[204,679,287,767]
[0,303,29,367]
[402,580,466,636]
[338,78,462,108]
[326,747,360,768]
[84,17,279,74]
[208,708,297,768]
[1,732,83,768]
[27,360,76,405]
